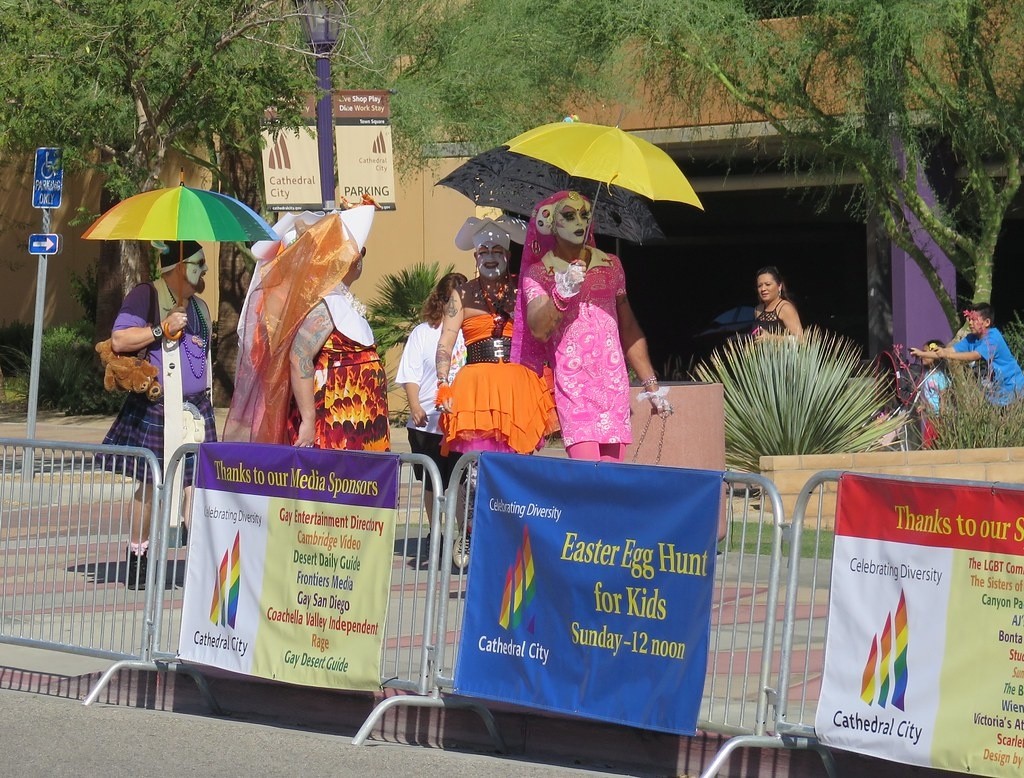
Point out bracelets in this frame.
[552,288,570,311]
[437,377,449,388]
[151,324,165,340]
[642,376,657,386]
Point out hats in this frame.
[150,240,204,274]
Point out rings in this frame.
[439,406,445,410]
[183,317,187,321]
[435,406,439,411]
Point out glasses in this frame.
[184,258,206,267]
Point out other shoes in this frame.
[426,533,444,559]
[126,546,147,590]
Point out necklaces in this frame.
[478,276,511,309]
[340,284,367,317]
[169,285,209,378]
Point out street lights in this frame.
[292,1,343,216]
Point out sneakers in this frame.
[453,536,471,567]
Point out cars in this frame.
[664,296,761,381]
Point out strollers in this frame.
[845,343,940,454]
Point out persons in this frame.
[95,241,218,590]
[222,204,392,453]
[752,266,804,345]
[510,191,673,462]
[909,303,1024,412]
[917,339,953,449]
[436,217,560,569]
[395,272,467,557]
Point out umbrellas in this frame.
[435,145,667,247]
[504,122,705,262]
[81,169,284,340]
